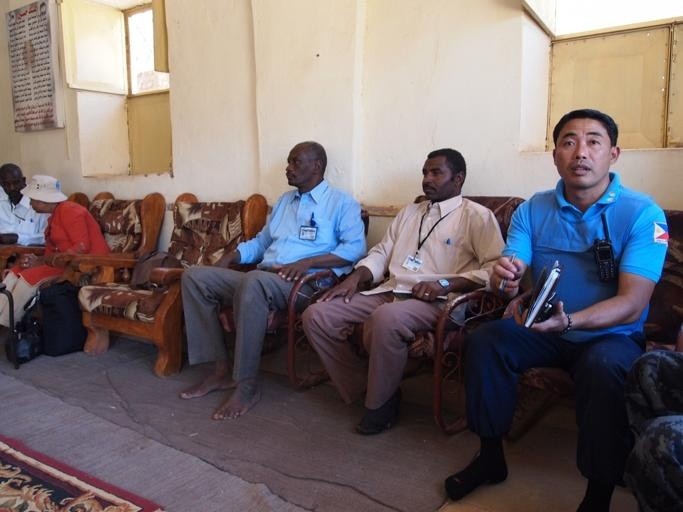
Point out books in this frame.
[523,259,565,329]
[393,276,448,301]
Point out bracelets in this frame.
[553,314,573,337]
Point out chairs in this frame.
[70,193,267,379]
[0,191,166,357]
[302,195,525,436]
[217,210,368,392]
[462,207,683,443]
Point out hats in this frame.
[20,175,68,203]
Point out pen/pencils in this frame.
[311,212,315,227]
[498,253,515,292]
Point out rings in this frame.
[424,292,429,296]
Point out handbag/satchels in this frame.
[41,280,87,357]
[6,319,43,364]
[132,249,184,289]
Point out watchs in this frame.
[438,278,449,294]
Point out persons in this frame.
[446,112,671,511]
[180,141,367,420]
[0,164,51,263]
[624,350,682,511]
[301,147,505,435]
[0,174,111,329]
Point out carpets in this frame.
[0,435,162,512]
